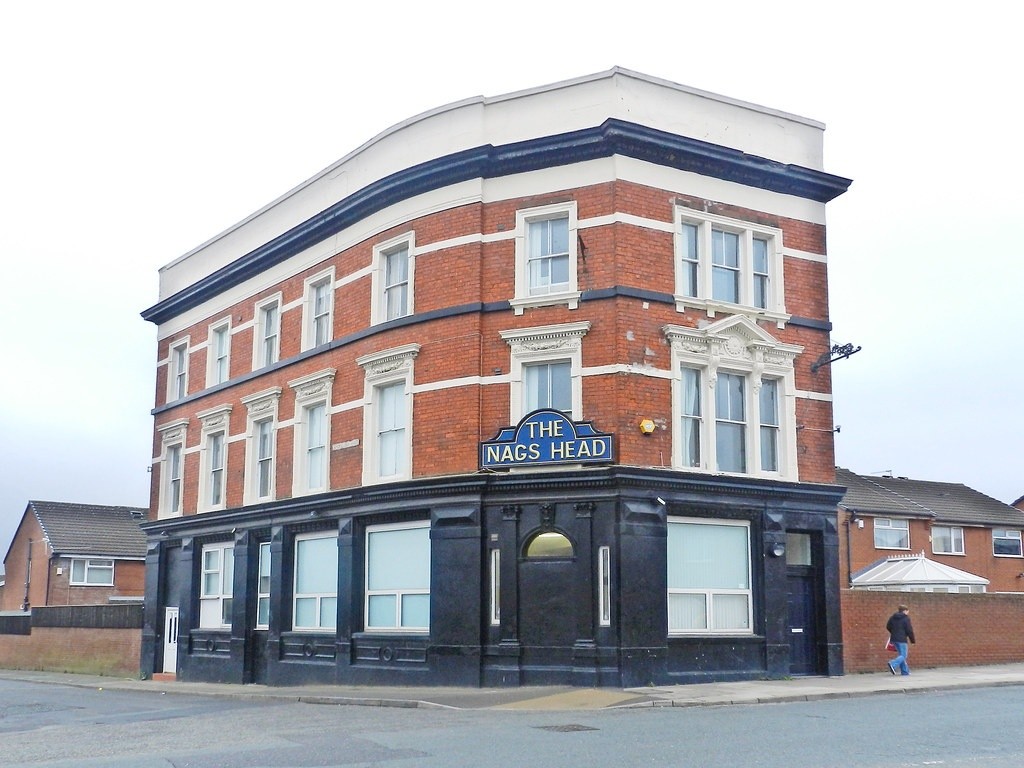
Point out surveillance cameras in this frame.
[858,519,864,529]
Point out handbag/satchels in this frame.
[885,637,897,652]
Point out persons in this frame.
[886,605,915,675]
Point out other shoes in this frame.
[907,672,911,675]
[888,662,896,675]
